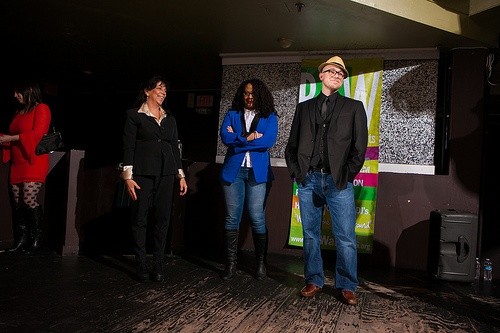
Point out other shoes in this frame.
[135,266,150,282]
[154,269,163,282]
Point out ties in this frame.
[320,97,329,120]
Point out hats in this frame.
[318,55,349,79]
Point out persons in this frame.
[218,78,278,281]
[118,77,188,281]
[284,56,369,304]
[0,78,52,254]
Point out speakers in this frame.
[427,209,480,283]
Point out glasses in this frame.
[323,68,344,77]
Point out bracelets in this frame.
[255,131,258,138]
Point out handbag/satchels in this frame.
[36,126,64,155]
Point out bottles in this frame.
[483,258,492,281]
[474,258,481,279]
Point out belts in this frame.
[309,167,331,175]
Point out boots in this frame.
[220,231,240,281]
[9,203,29,252]
[251,230,268,280]
[24,201,45,255]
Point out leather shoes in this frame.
[341,289,357,305]
[300,283,323,297]
